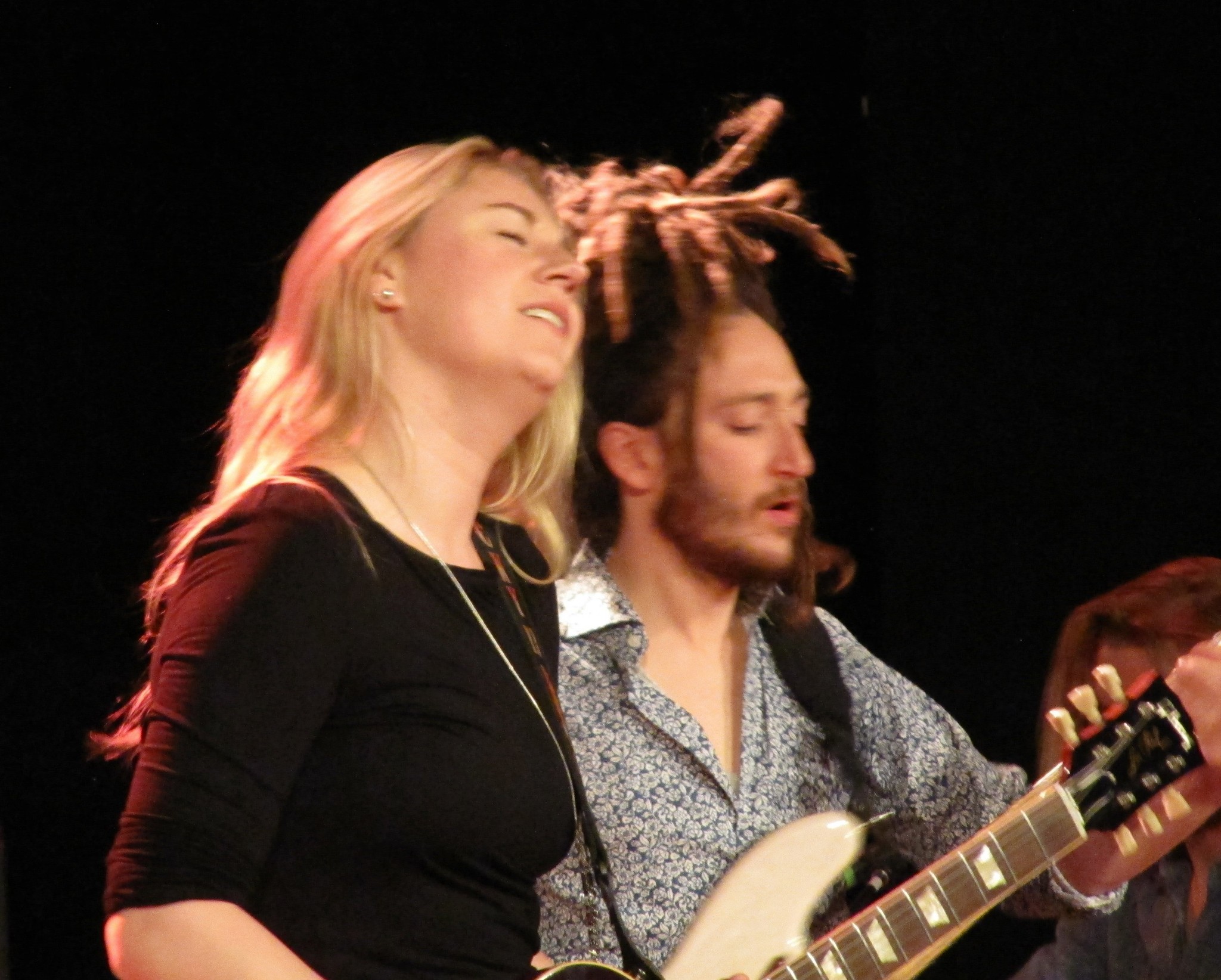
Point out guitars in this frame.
[537,660,1208,980]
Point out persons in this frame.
[534,255,1220,980]
[88,137,589,980]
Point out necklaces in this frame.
[333,430,595,901]
